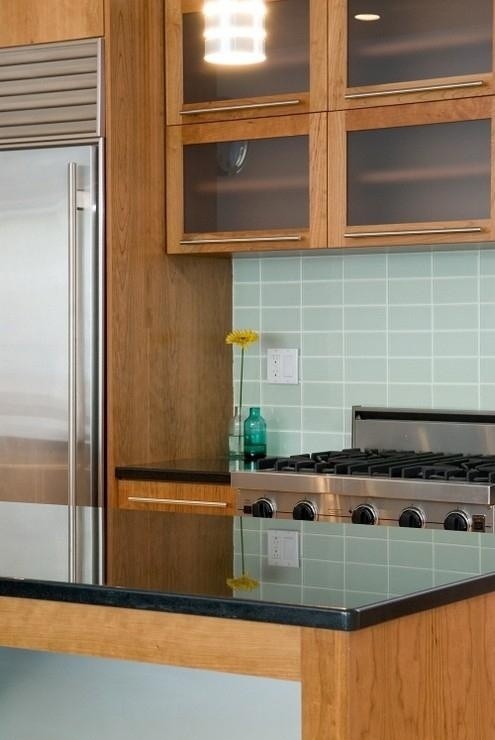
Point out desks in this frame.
[1,498,494,740]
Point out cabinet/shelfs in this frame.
[114,480,238,514]
[327,1,495,248]
[159,1,327,255]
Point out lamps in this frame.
[200,1,268,68]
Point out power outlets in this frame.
[266,529,300,569]
[265,348,299,384]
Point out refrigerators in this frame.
[1,144,101,501]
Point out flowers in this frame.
[223,328,259,453]
[224,516,261,593]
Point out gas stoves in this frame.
[231,445,495,534]
